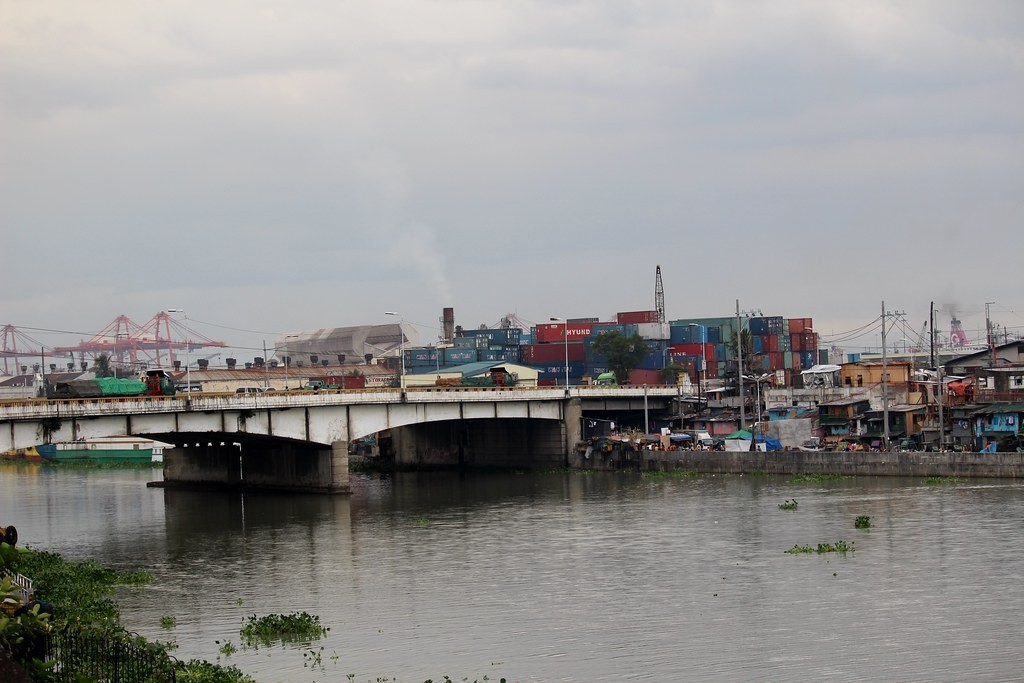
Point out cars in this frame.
[262,387,277,397]
[641,430,724,451]
[803,436,871,452]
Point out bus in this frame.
[173,382,202,392]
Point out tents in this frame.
[723,429,783,453]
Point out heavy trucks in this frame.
[597,372,632,389]
[56,370,176,404]
[435,367,513,391]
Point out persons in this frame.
[818,439,826,449]
[576,441,725,452]
[756,446,762,452]
[845,444,851,452]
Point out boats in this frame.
[34,433,176,458]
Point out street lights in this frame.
[740,372,775,435]
[385,312,407,404]
[284,335,299,390]
[550,317,568,390]
[643,383,649,434]
[689,324,707,392]
[167,309,190,393]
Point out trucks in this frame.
[304,380,340,394]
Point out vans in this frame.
[235,387,264,396]
